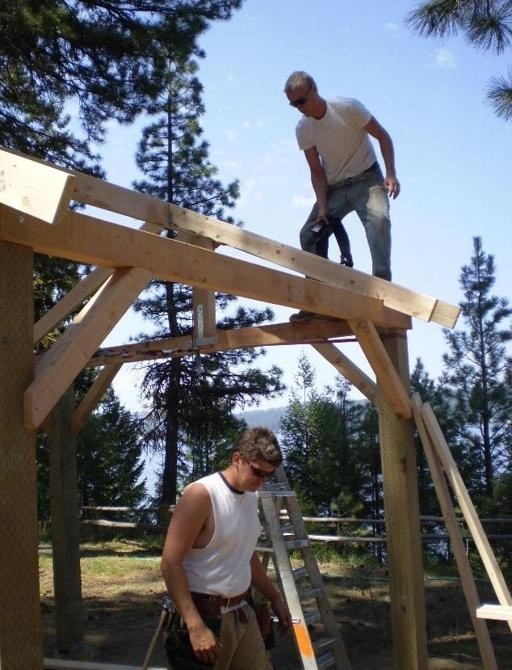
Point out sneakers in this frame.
[289,309,316,322]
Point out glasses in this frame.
[289,83,313,107]
[246,459,276,477]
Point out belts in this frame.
[192,586,252,608]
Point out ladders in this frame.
[142,459,353,670]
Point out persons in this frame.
[160,427,292,670]
[283,70,400,324]
